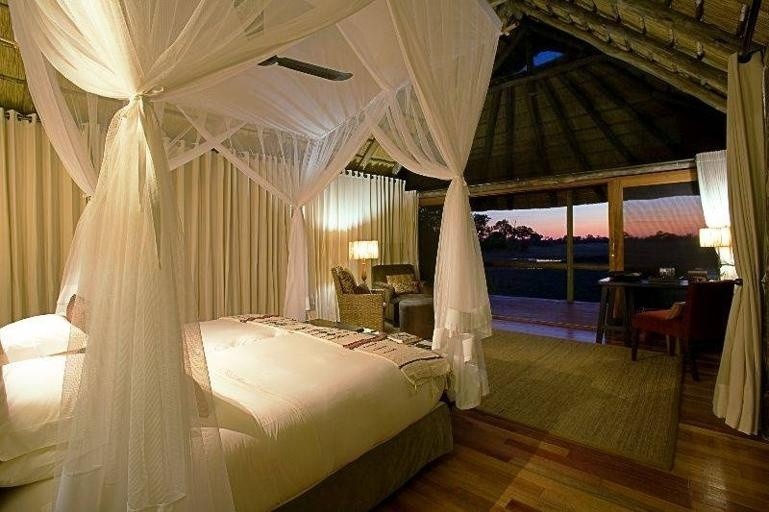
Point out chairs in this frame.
[662,247,720,279]
[632,280,734,381]
[330,264,425,335]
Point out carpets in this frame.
[440,327,684,471]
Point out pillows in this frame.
[0,314,101,487]
[666,302,685,321]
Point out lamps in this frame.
[699,227,732,282]
[349,240,379,288]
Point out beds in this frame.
[0,314,452,512]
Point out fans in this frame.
[233,1,353,81]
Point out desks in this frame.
[399,297,433,340]
[596,276,737,343]
